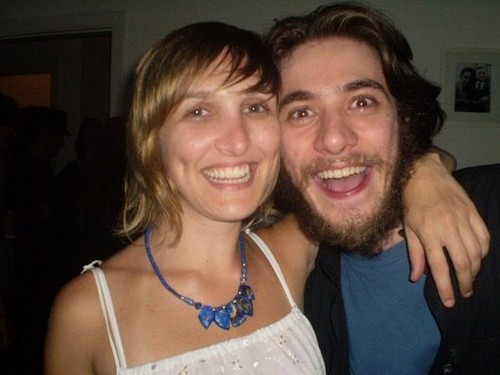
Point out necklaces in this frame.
[144,223,255,330]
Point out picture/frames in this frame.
[437,46,500,128]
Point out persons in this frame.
[43,20,490,375]
[265,3,500,375]
